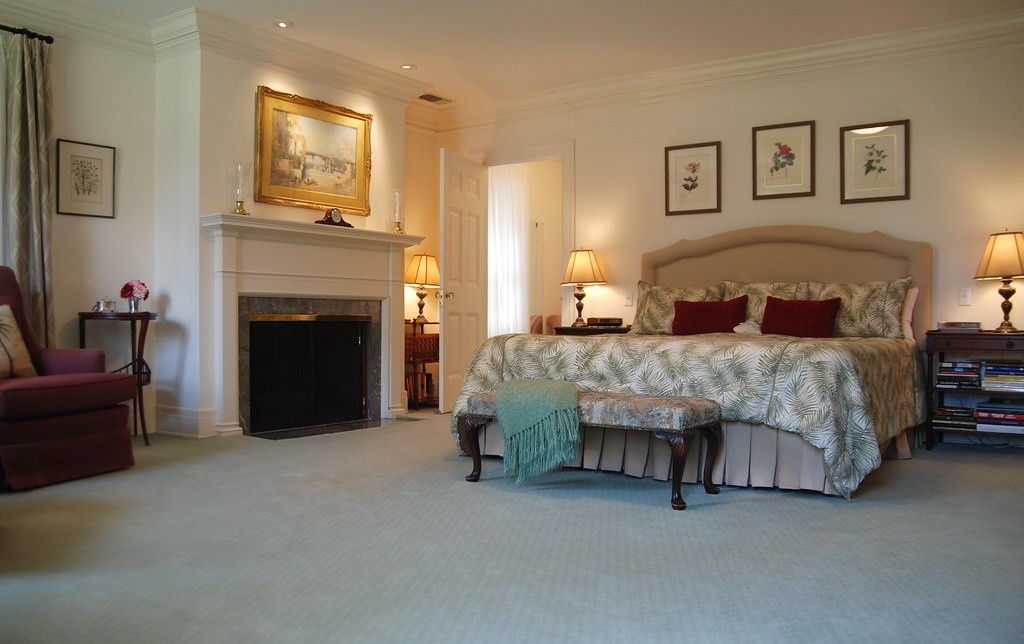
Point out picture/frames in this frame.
[56,137,117,218]
[840,119,911,203]
[253,84,373,217]
[664,140,722,216]
[752,120,816,201]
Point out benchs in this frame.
[464,377,724,512]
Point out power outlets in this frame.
[958,287,971,306]
[624,290,633,306]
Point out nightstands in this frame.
[554,326,630,336]
[923,329,1024,451]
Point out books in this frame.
[938,321,980,333]
[931,357,1024,434]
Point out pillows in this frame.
[900,287,919,340]
[807,274,913,340]
[760,296,842,338]
[627,280,725,335]
[672,295,748,335]
[0,304,38,379]
[721,279,810,325]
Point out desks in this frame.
[404,321,440,410]
[78,311,158,446]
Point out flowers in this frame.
[120,280,150,301]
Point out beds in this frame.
[450,225,931,503]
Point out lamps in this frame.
[973,226,1024,333]
[405,252,441,322]
[559,246,608,327]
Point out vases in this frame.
[127,296,139,313]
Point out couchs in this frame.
[0,265,139,495]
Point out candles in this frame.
[231,159,254,215]
[391,188,407,235]
